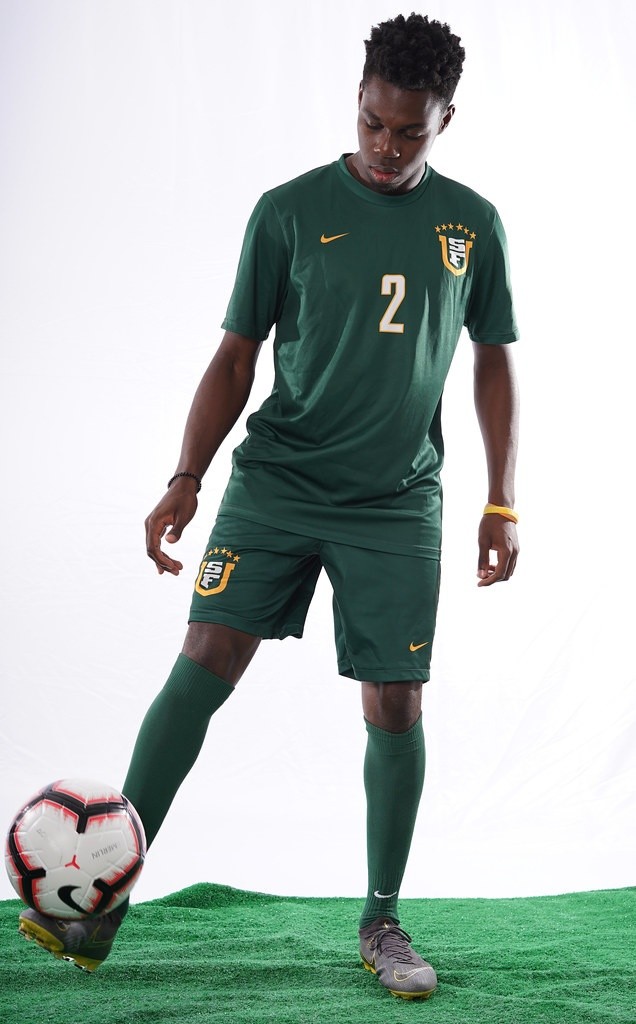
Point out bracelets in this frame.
[482,502,520,524]
[167,471,203,494]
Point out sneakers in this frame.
[15,907,120,974]
[358,916,438,999]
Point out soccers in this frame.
[4,778,147,920]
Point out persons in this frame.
[17,12,521,1005]
